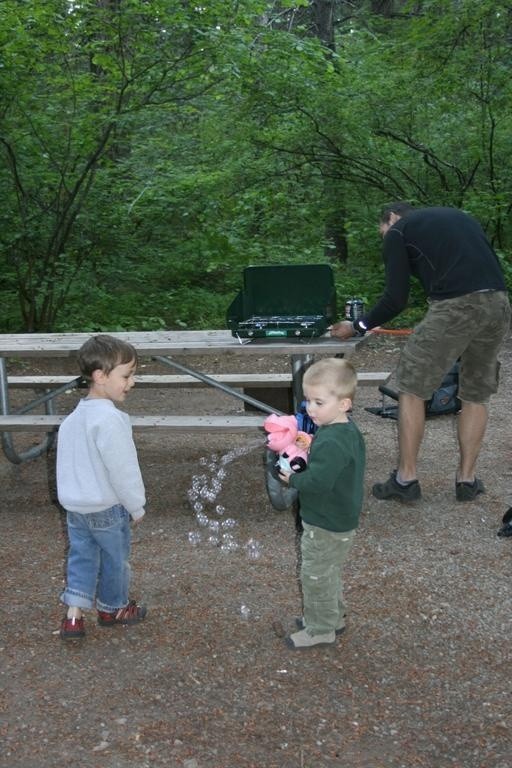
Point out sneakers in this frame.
[455,473,486,502]
[285,613,348,651]
[97,597,148,627]
[372,468,421,502]
[59,617,87,640]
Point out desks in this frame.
[0,330,381,511]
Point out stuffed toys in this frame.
[263,414,313,475]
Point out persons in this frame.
[278,359,369,647]
[44,331,153,640]
[327,200,511,502]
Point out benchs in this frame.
[2,414,299,510]
[7,369,391,442]
